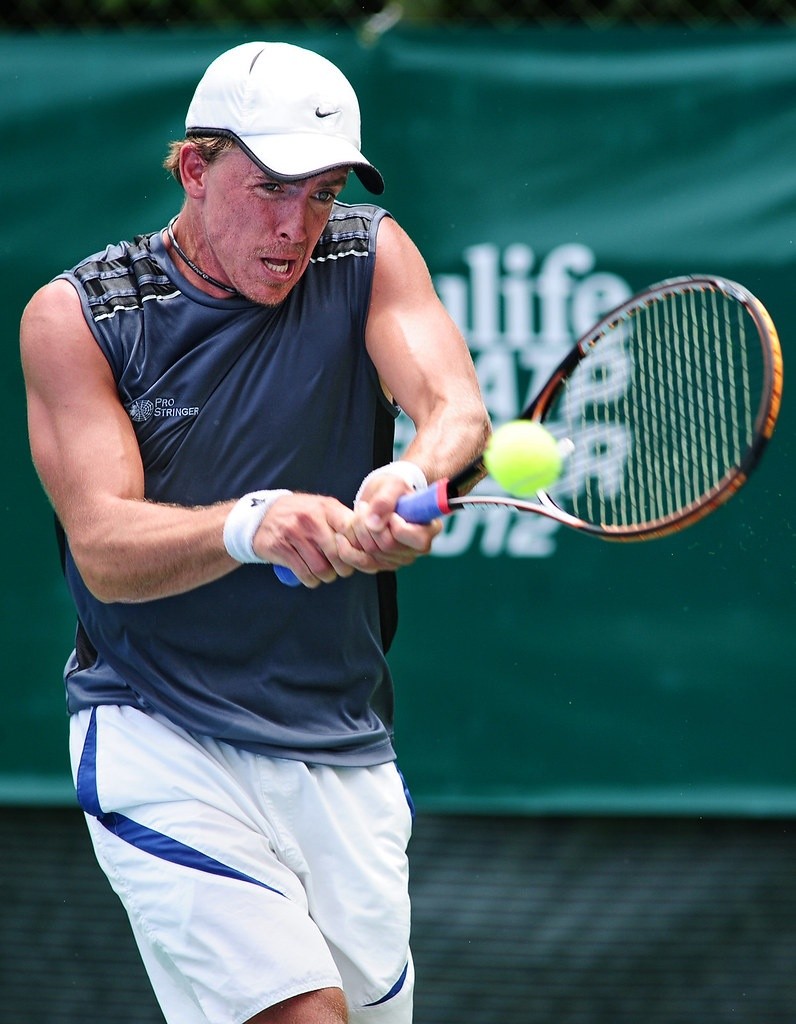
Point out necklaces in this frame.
[167,215,237,293]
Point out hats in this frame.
[183,41,385,197]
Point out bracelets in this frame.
[223,488,291,564]
[354,460,427,507]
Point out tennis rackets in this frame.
[270,273,784,589]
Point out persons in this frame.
[22,42,492,1024]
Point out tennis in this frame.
[482,418,562,495]
